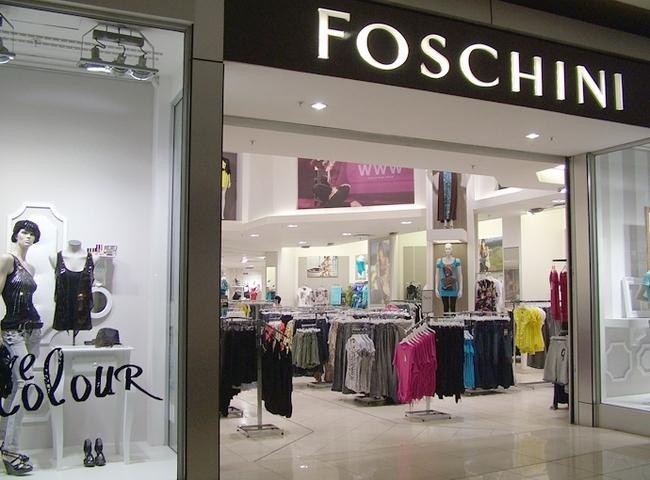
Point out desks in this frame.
[53,345,135,465]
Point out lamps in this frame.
[0,13,15,63]
[76,22,159,81]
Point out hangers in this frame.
[551,261,556,271]
[260,307,511,352]
[560,261,567,271]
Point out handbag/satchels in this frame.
[95,328,119,346]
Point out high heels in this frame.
[0,442,29,462]
[2,450,33,475]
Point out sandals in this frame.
[94,439,106,466]
[84,440,95,467]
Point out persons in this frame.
[371,243,390,303]
[356,255,367,280]
[0,220,41,476]
[48,239,97,330]
[435,242,463,316]
[309,158,351,207]
[319,256,332,276]
[221,158,232,219]
[479,239,490,273]
[221,277,257,300]
[273,295,282,307]
[433,169,458,228]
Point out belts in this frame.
[11,320,43,331]
